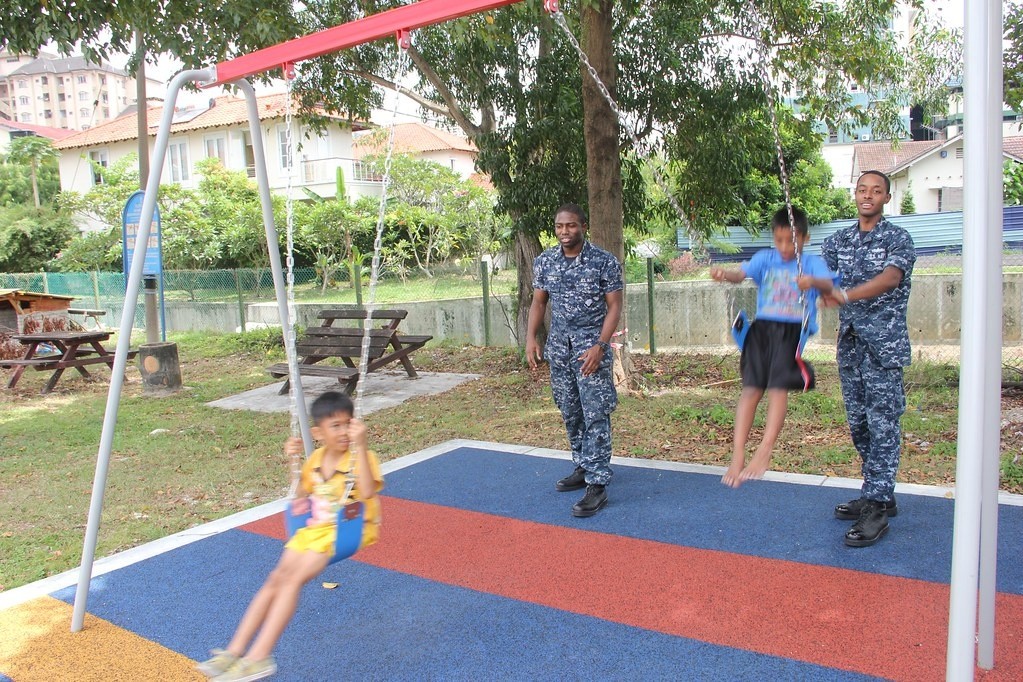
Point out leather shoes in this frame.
[554,465,587,491]
[833,494,898,519]
[844,502,890,547]
[572,484,609,517]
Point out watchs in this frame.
[596,340,611,352]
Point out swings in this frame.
[276,39,413,567]
[544,0,815,391]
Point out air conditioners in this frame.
[862,134,869,141]
[1016,115,1023,121]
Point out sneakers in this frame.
[210,657,277,682]
[197,649,241,678]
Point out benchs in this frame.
[0,359,64,395]
[341,334,434,378]
[76,347,139,382]
[264,362,360,399]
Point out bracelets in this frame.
[840,288,850,304]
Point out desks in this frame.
[279,310,421,398]
[8,332,128,395]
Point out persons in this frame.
[820,171,917,548]
[526,202,624,517]
[710,206,840,488]
[196,392,383,682]
